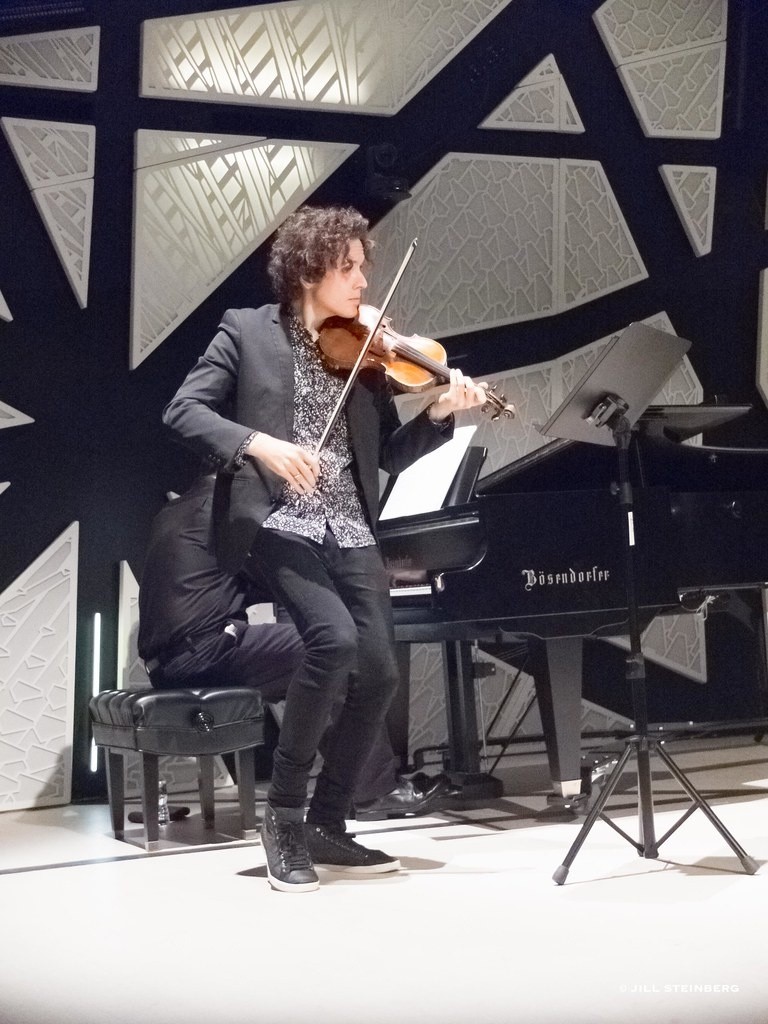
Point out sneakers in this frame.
[259,802,319,892]
[303,820,400,874]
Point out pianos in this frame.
[275,393,768,823]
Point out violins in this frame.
[317,303,517,423]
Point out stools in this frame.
[90,686,265,852]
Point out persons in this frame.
[163,206,487,892]
[139,454,450,821]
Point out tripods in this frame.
[535,322,756,883]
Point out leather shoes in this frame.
[354,774,451,820]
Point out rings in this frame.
[294,473,301,479]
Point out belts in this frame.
[144,612,248,675]
